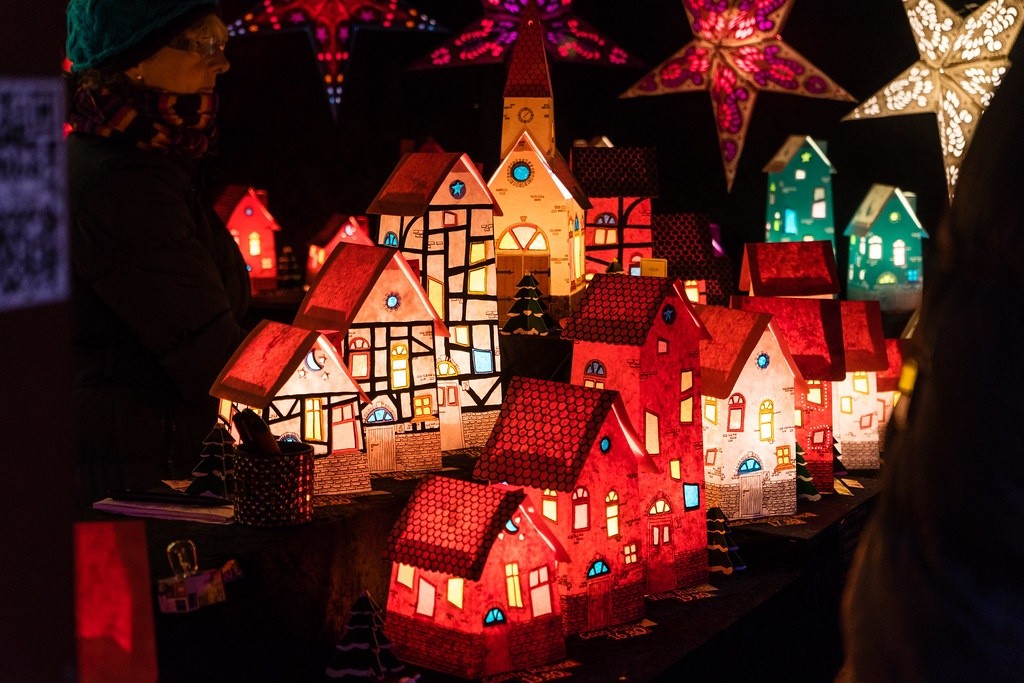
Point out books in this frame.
[92,496,236,525]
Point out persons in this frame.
[829,21,1024,683]
[57,0,255,511]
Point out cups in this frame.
[232,441,314,525]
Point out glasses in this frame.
[168,37,224,61]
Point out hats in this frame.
[64,0,217,78]
